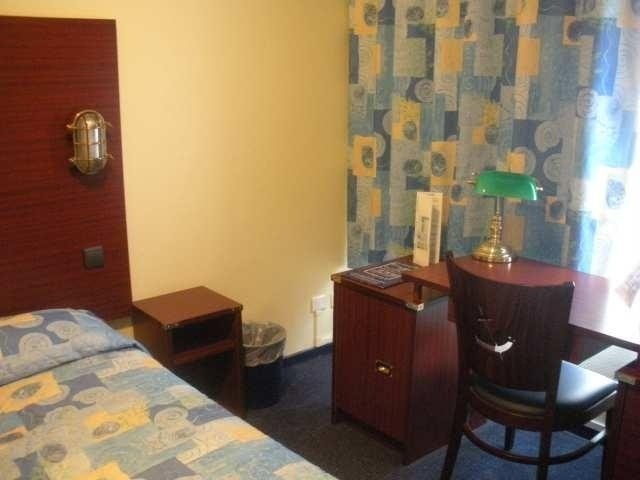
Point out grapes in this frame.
[132,286,248,420]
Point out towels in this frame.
[243,323,287,409]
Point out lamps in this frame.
[439,252,617,480]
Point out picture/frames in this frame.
[327,249,640,480]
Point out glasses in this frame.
[467,171,545,264]
[66,109,115,177]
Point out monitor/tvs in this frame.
[341,253,418,289]
[413,192,445,268]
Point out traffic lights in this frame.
[0,304,142,386]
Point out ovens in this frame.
[1,304,338,480]
